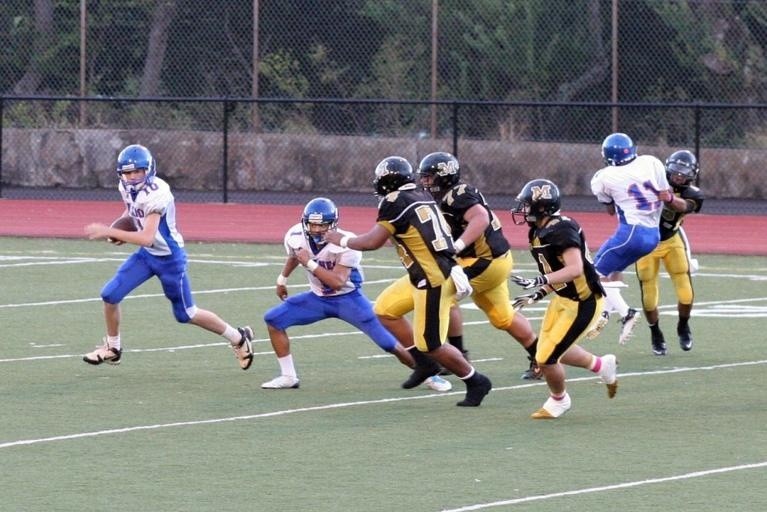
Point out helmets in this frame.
[371,155,416,196]
[665,150,701,189]
[600,131,637,167]
[301,198,339,245]
[512,177,561,231]
[416,151,461,198]
[116,143,157,194]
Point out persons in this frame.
[416,150,546,380]
[585,132,672,346]
[508,177,619,420]
[323,155,492,407]
[635,150,706,356]
[259,196,453,393]
[80,144,256,371]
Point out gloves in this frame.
[512,288,550,313]
[510,274,550,291]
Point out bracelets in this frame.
[306,258,320,274]
[664,193,675,204]
[453,239,466,255]
[340,235,349,249]
[275,274,288,286]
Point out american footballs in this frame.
[109,217,142,242]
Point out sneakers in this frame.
[521,356,545,380]
[231,325,256,370]
[456,374,493,407]
[585,311,610,341]
[530,393,572,420]
[439,350,470,377]
[599,354,620,399]
[401,358,442,392]
[425,374,453,392]
[260,375,301,389]
[617,307,641,345]
[82,342,124,366]
[677,321,694,351]
[651,328,668,356]
